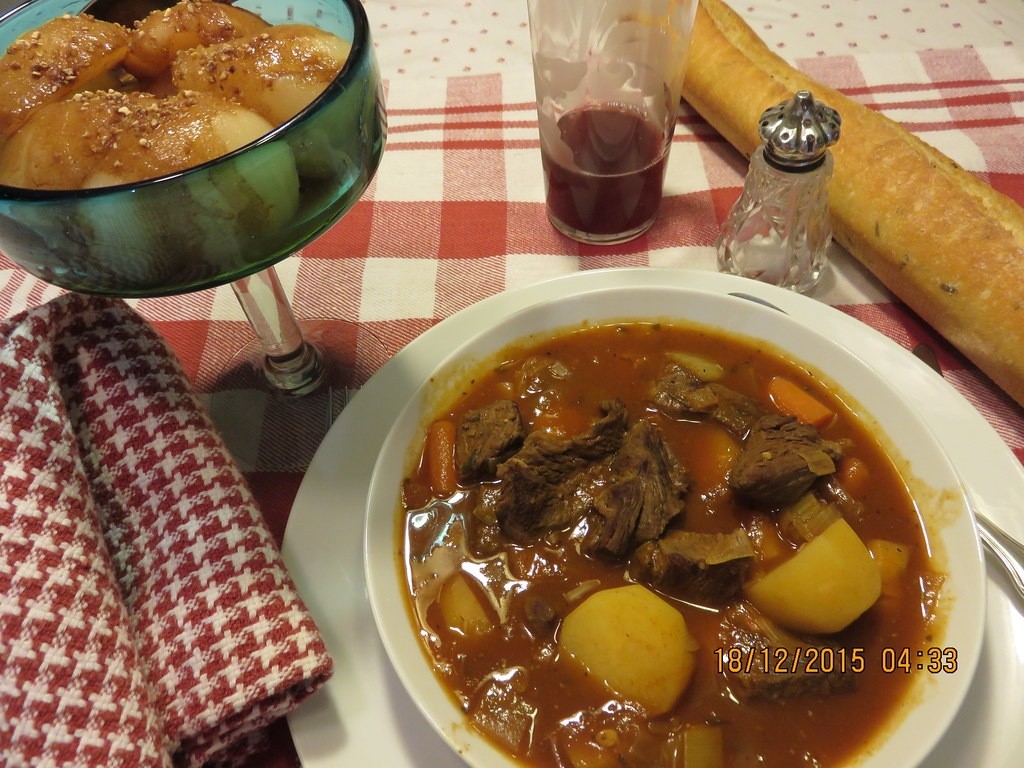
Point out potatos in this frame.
[559,587,692,713]
[750,519,882,631]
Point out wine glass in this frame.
[0,0,388,470]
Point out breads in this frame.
[615,0,1024,408]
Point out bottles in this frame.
[717,92,842,296]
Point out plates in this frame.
[365,288,984,768]
[284,266,1024,768]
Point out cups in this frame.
[527,0,701,245]
